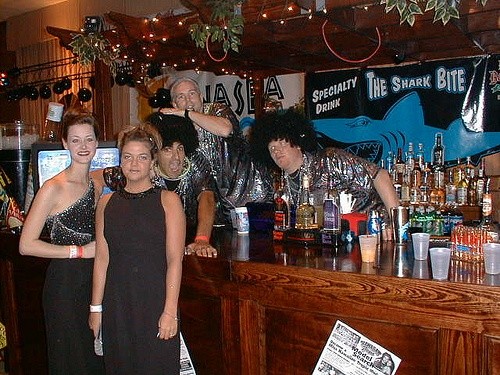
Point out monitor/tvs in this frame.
[31,142,123,198]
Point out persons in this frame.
[18,112,102,375]
[87,126,186,375]
[89,77,400,257]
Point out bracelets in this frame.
[69,245,82,259]
[196,236,209,241]
[163,309,178,321]
[89,305,102,312]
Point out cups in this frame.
[359,235,377,263]
[392,205,410,244]
[429,247,451,280]
[483,243,500,275]
[236,234,249,262]
[412,233,430,261]
[361,262,377,275]
[394,242,412,278]
[235,207,249,234]
[412,259,429,280]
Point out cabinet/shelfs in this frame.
[393,162,483,240]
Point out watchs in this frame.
[185,106,195,120]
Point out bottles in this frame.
[368,133,500,242]
[271,173,341,235]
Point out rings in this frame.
[170,331,175,337]
